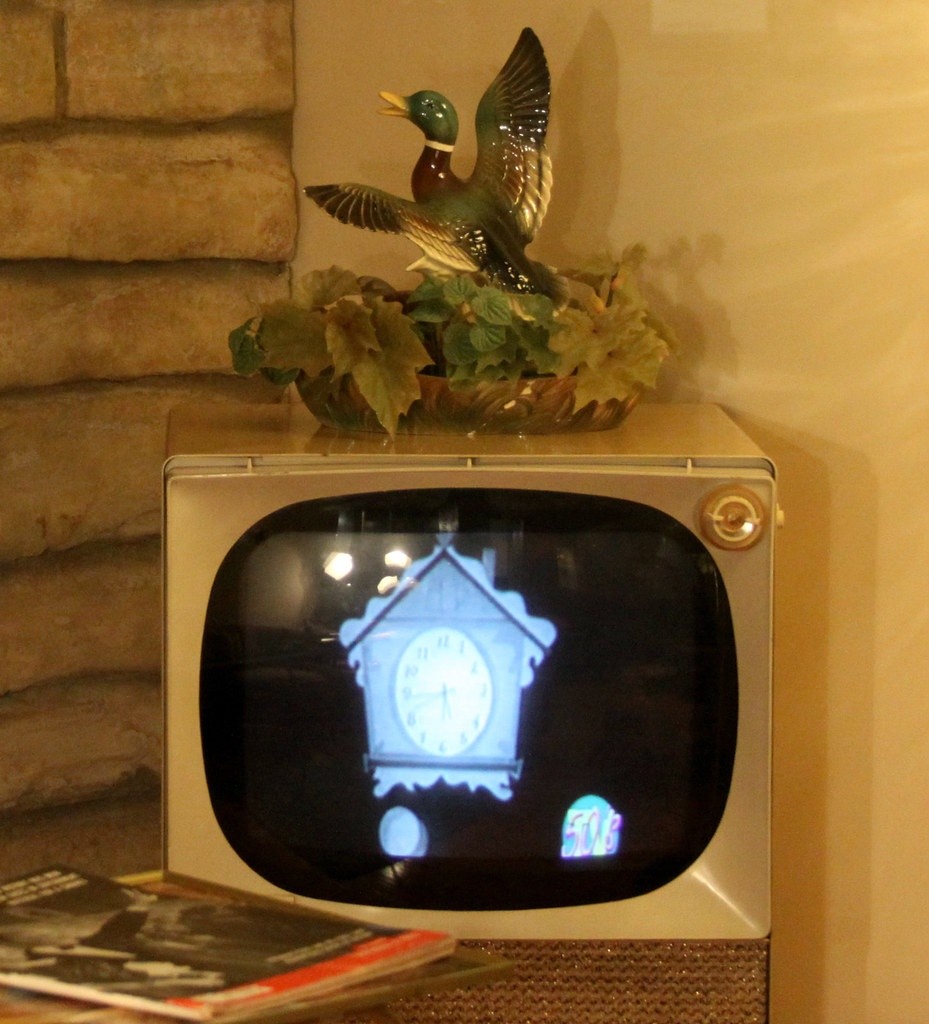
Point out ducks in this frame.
[303,25,572,325]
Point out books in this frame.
[0,862,514,1024]
